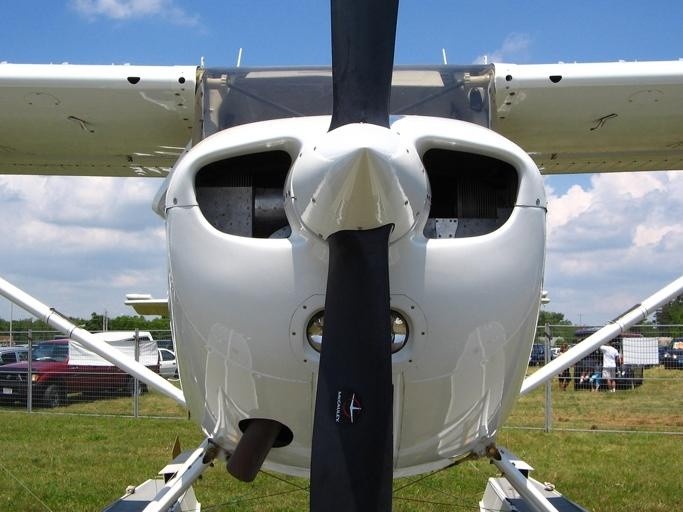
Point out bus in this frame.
[574,329,645,390]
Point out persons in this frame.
[590,361,602,392]
[615,348,628,375]
[600,340,618,392]
[557,343,572,393]
[580,368,593,384]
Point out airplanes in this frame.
[0,0,683,512]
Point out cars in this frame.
[659,337,683,368]
[529,344,560,366]
[0,332,178,407]
